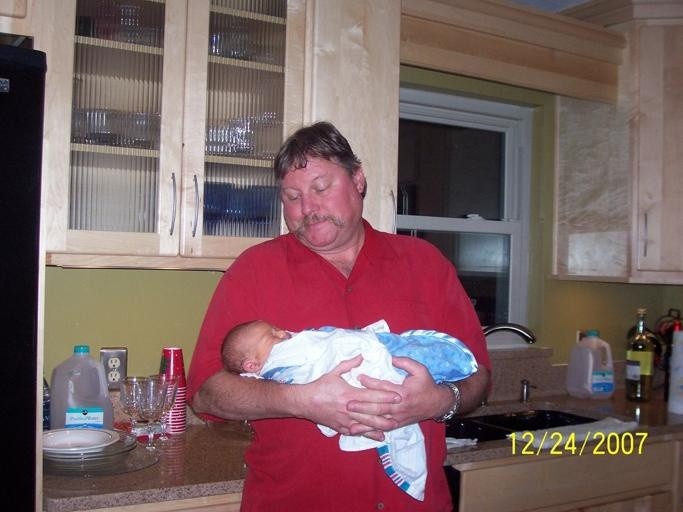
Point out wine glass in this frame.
[120,376,179,457]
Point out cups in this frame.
[158,346,188,435]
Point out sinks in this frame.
[471,407,600,432]
[444,416,518,443]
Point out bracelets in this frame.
[433,381,461,423]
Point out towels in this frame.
[506,417,638,451]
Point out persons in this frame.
[221,318,478,389]
[184,123,492,512]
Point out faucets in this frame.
[482,321,537,345]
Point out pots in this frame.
[654,308,683,344]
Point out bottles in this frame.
[667,320,683,415]
[624,307,656,403]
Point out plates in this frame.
[43,427,160,479]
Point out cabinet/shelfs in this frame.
[12,0,307,271]
[551,26,683,285]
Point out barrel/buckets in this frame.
[566,328,616,399]
[50,345,115,430]
